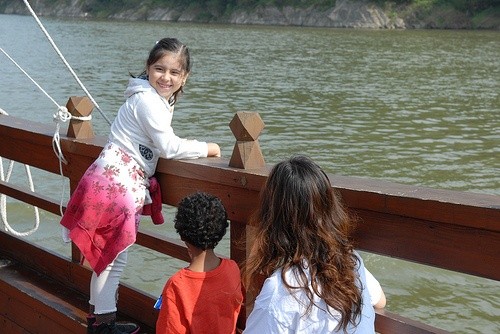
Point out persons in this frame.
[242,157,386,334]
[77,37,220,334]
[154,193,242,334]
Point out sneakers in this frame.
[91,323,128,334]
[86,314,140,334]
